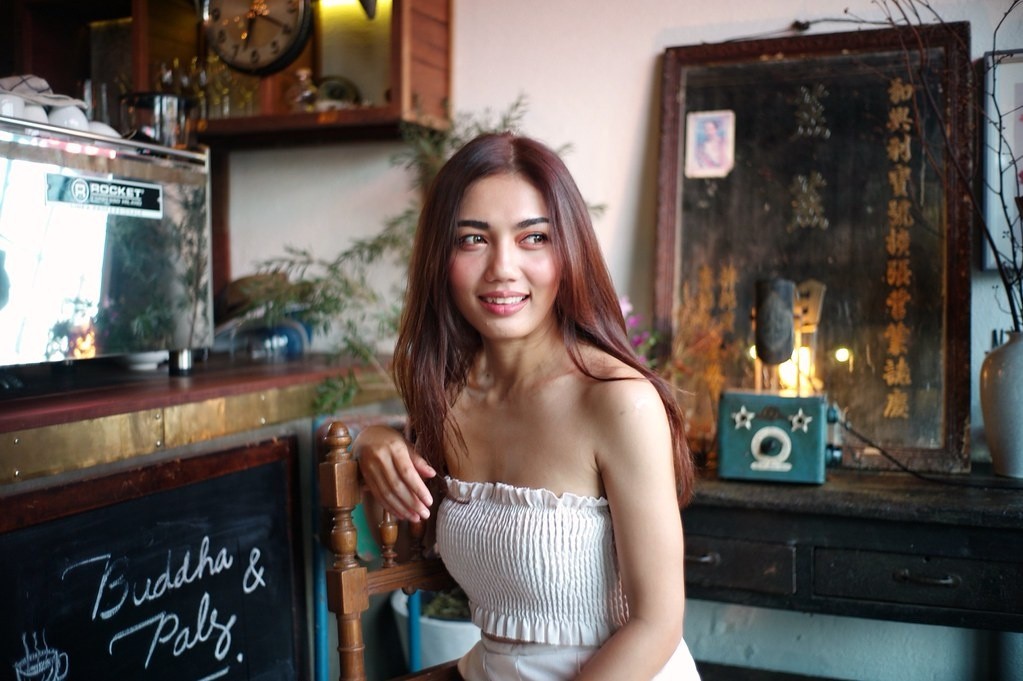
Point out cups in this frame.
[150,92,190,153]
[83,80,122,134]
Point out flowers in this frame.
[617,294,682,381]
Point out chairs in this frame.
[319,420,462,681]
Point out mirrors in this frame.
[650,19,974,474]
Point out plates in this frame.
[318,75,365,115]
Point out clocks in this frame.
[201,0,321,120]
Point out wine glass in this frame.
[151,46,254,118]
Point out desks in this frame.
[676,459,1023,636]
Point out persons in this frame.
[346,131,702,681]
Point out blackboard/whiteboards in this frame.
[0,431,311,681]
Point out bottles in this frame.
[285,67,320,115]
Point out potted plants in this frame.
[238,104,518,670]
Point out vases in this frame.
[979,330,1023,479]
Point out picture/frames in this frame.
[979,49,1023,272]
